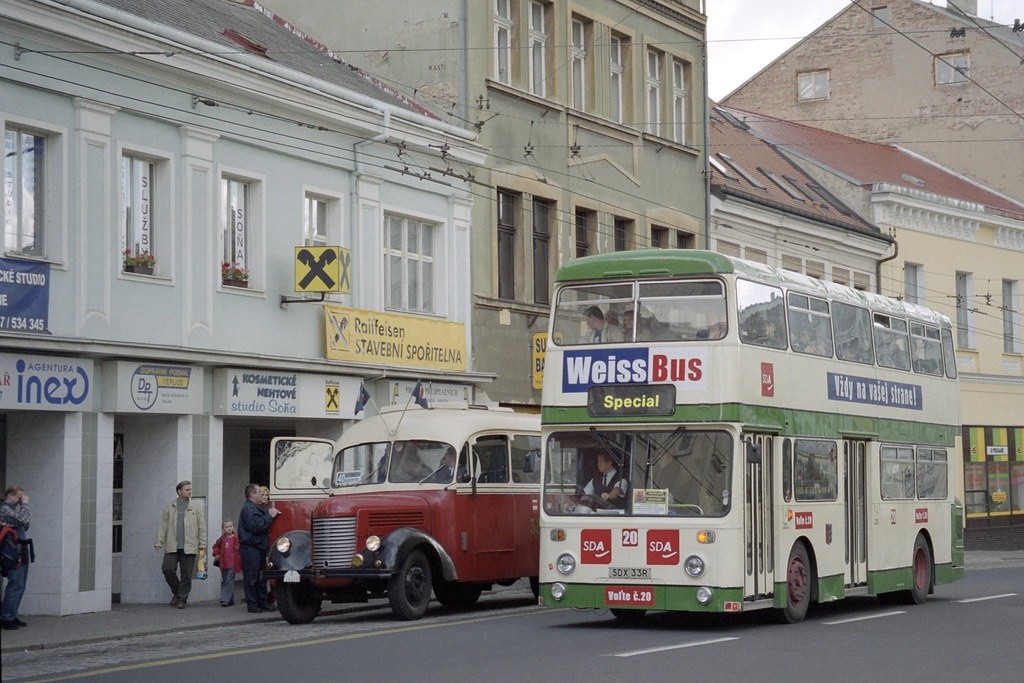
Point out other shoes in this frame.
[14,618,27,627]
[177,599,186,608]
[248,605,263,613]
[0,620,19,630]
[262,603,275,612]
[221,601,229,606]
[170,597,178,606]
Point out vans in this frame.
[268,401,577,622]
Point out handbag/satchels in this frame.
[0,522,23,567]
[196,547,209,580]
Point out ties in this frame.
[603,474,608,486]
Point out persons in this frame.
[238,483,281,614]
[578,299,953,377]
[377,441,506,483]
[0,486,36,630]
[213,519,243,607]
[154,480,208,609]
[580,449,629,516]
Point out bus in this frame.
[539,248,967,624]
